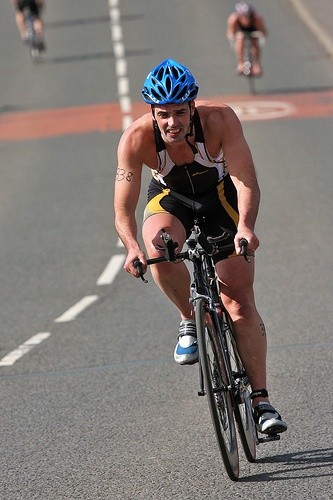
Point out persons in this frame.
[226,0,267,77]
[113,58,287,435]
[11,0,46,51]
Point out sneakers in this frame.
[252,403,287,433]
[174,321,198,364]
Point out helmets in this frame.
[141,59,199,105]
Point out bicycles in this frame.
[132,219,282,482]
[231,36,264,94]
[15,2,45,59]
